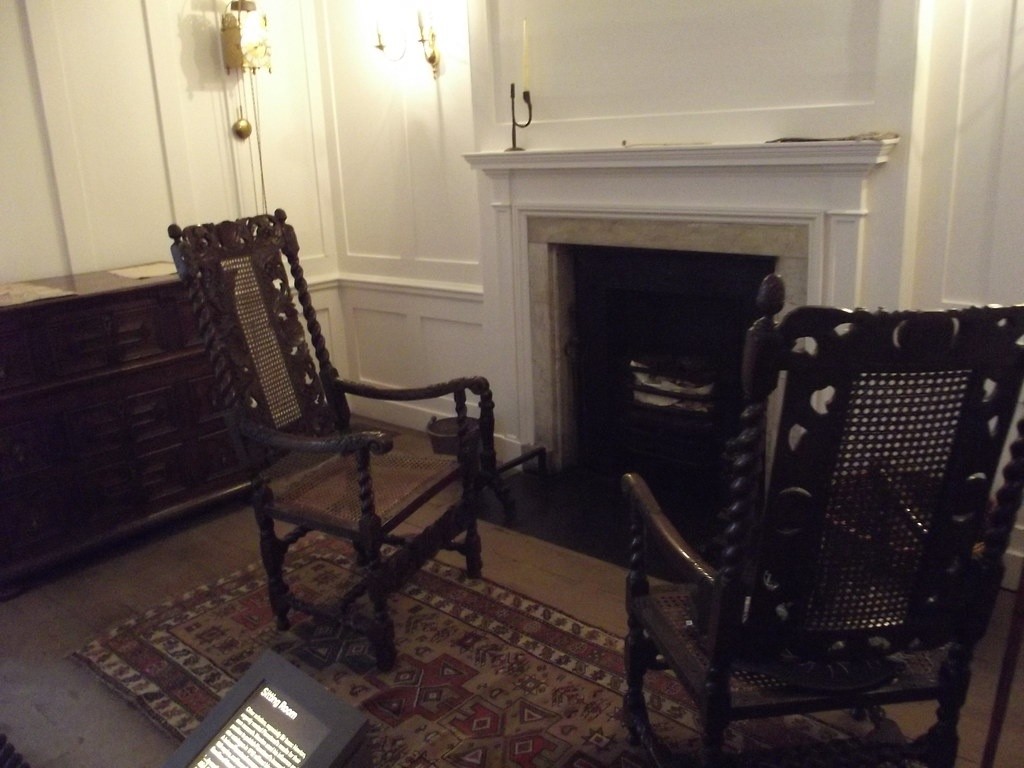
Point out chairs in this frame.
[167,207,497,670]
[617,272,1024,768]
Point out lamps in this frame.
[221,0,277,140]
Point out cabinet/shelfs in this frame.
[0,262,253,602]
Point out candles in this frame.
[523,18,530,92]
[418,12,423,29]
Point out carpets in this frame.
[73,528,931,768]
